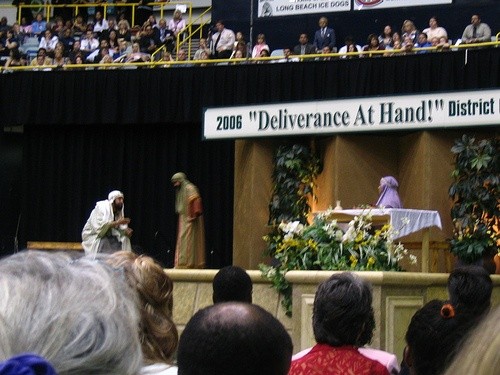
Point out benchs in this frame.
[27,241,82,251]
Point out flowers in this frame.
[262,201,421,274]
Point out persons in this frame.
[401,264,499,375]
[458,14,491,47]
[193,15,450,54]
[290,270,390,375]
[0,248,178,375]
[171,171,205,270]
[0,0,195,68]
[177,301,293,374]
[81,190,134,253]
[373,175,402,208]
[211,265,253,303]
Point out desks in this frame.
[313,208,442,272]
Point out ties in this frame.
[215,32,220,53]
[473,27,476,38]
[162,30,164,37]
[321,30,325,39]
[302,46,304,54]
